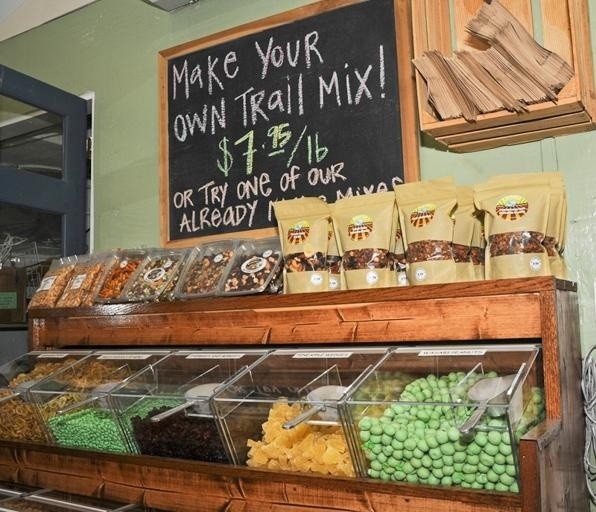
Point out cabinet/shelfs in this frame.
[411,0,596,156]
[0,274,588,512]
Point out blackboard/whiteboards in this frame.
[158,1,420,247]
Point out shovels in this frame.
[0,379,52,404]
[152,383,233,422]
[459,373,523,436]
[282,385,349,428]
[54,381,125,413]
